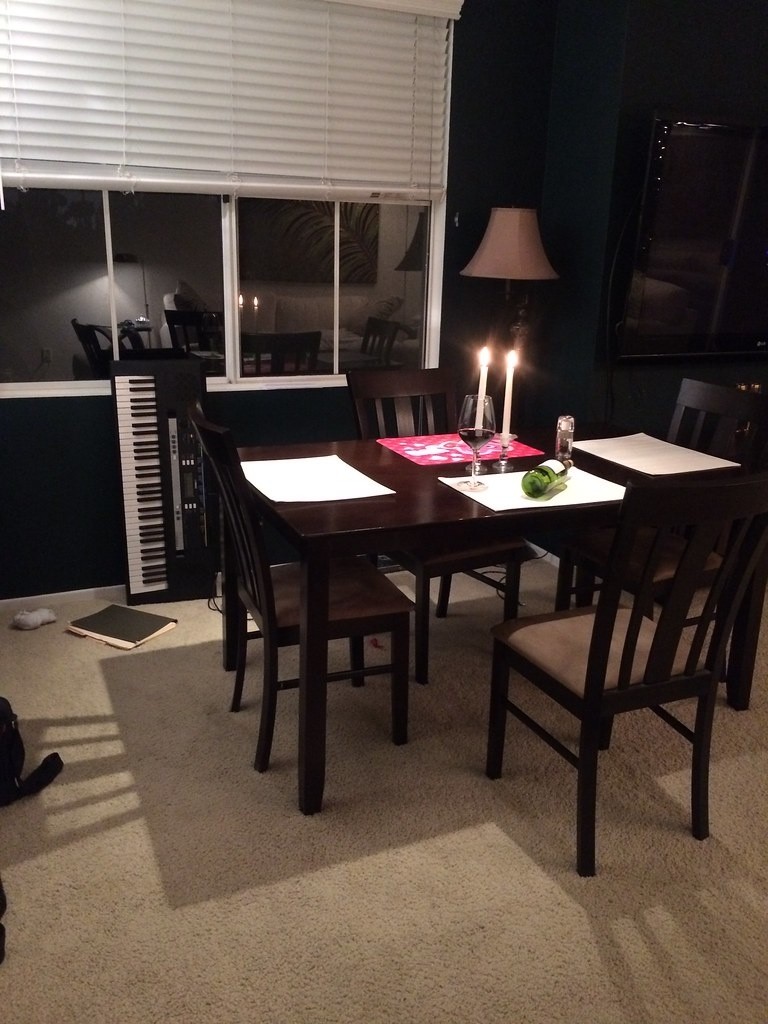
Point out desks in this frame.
[237,422,768,815]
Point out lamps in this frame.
[457,208,559,359]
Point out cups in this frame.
[555,415,575,458]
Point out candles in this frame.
[474,346,492,428]
[239,294,244,331]
[500,349,518,437]
[253,295,259,334]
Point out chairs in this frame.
[211,330,321,377]
[163,310,225,350]
[484,471,768,877]
[71,319,108,379]
[360,316,401,368]
[347,366,537,684]
[188,406,416,772]
[553,378,768,683]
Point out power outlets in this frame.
[42,347,52,363]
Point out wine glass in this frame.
[457,395,496,492]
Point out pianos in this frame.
[109,359,218,607]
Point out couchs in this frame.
[174,279,405,365]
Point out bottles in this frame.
[522,458,574,498]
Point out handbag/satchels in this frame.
[0,696,64,807]
